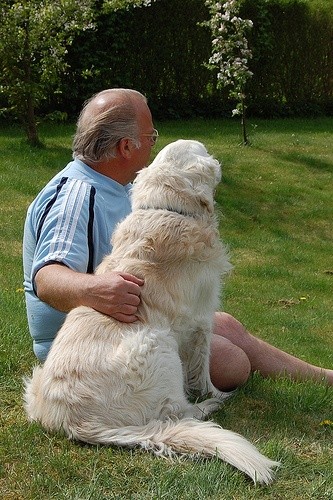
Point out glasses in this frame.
[133,129,159,146]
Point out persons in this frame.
[23,89,333,393]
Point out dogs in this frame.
[20,139,281,487]
